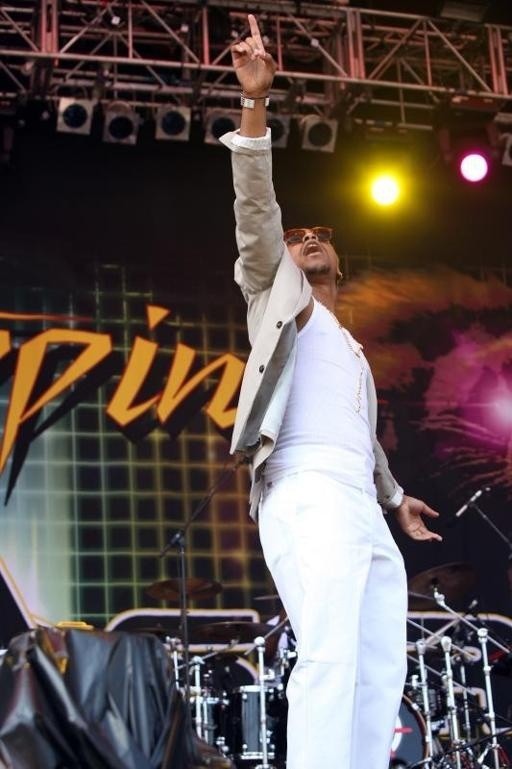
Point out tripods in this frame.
[414,629,512,769]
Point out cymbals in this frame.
[147,576,222,603]
[407,560,473,597]
[201,622,281,644]
[253,593,280,602]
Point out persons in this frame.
[222,13,447,769]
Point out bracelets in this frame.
[239,92,270,110]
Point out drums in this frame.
[389,696,429,769]
[227,687,284,762]
[187,697,228,756]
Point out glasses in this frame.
[283,226,335,244]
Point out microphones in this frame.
[456,487,490,517]
